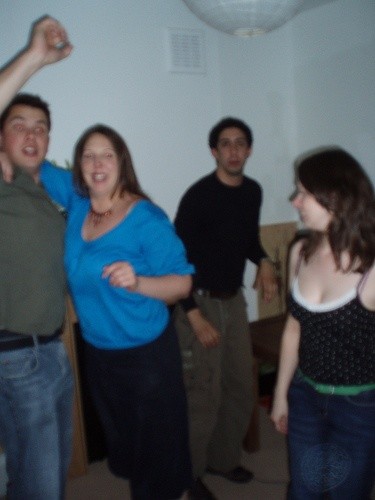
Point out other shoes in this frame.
[206,462,254,484]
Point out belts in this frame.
[0,321,67,351]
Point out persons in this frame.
[0,126,196,500]
[272,145,375,500]
[169,118,277,500]
[0,15,74,500]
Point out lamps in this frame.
[184,0,300,37]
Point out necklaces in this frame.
[89,191,121,229]
